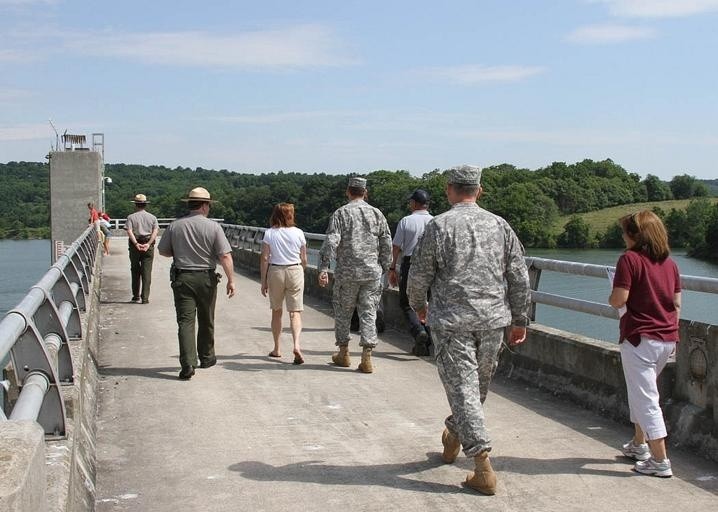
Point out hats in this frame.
[407,189,431,203]
[348,177,368,188]
[446,163,483,186]
[130,193,151,204]
[180,186,219,202]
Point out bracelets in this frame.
[135,242,138,247]
[389,268,395,270]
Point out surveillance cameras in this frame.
[105,177,112,186]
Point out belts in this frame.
[173,268,216,273]
[269,262,302,267]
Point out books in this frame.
[605,265,629,319]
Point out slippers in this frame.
[292,350,304,364]
[268,350,281,357]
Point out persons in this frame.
[406,165,532,496]
[97,216,111,232]
[122,194,160,303]
[261,202,307,364]
[157,187,237,378]
[88,202,102,242]
[318,176,393,372]
[606,210,682,477]
[388,189,434,356]
[88,217,112,257]
[351,304,385,332]
[96,212,111,224]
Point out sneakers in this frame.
[142,299,150,304]
[375,312,385,334]
[177,364,195,380]
[634,455,673,477]
[131,295,141,302]
[412,330,428,356]
[199,357,217,368]
[622,438,652,461]
[350,314,359,331]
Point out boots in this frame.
[332,340,351,368]
[465,448,497,495]
[358,344,374,373]
[441,426,461,464]
